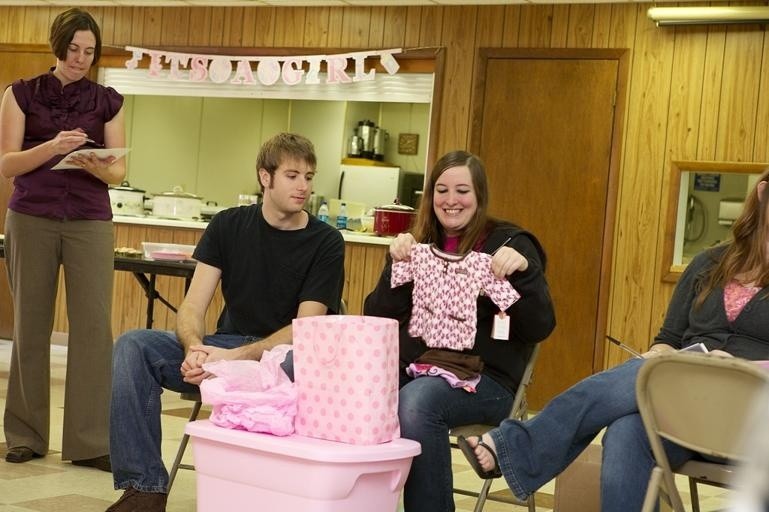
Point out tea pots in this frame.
[346,120,391,161]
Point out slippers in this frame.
[455,432,505,481]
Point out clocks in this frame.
[397,132,419,156]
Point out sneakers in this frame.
[104,486,168,511]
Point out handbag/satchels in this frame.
[293,315,400,444]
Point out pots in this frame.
[200,201,229,220]
[371,199,416,236]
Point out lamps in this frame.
[646,4,769,28]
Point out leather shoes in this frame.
[72,455,113,474]
[5,447,34,463]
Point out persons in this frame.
[0,7,129,472]
[457,168,768,511]
[361,148,557,511]
[101,131,347,512]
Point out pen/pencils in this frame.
[55,130,105,146]
[606,335,644,358]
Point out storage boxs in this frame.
[181,417,425,511]
[551,443,605,512]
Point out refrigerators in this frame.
[337,165,404,208]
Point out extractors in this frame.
[715,175,761,228]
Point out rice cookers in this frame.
[103,180,151,217]
[148,185,204,222]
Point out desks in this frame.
[0,238,222,334]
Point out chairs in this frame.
[445,334,544,512]
[633,345,769,512]
[156,295,351,495]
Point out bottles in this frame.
[317,201,329,225]
[335,203,347,229]
